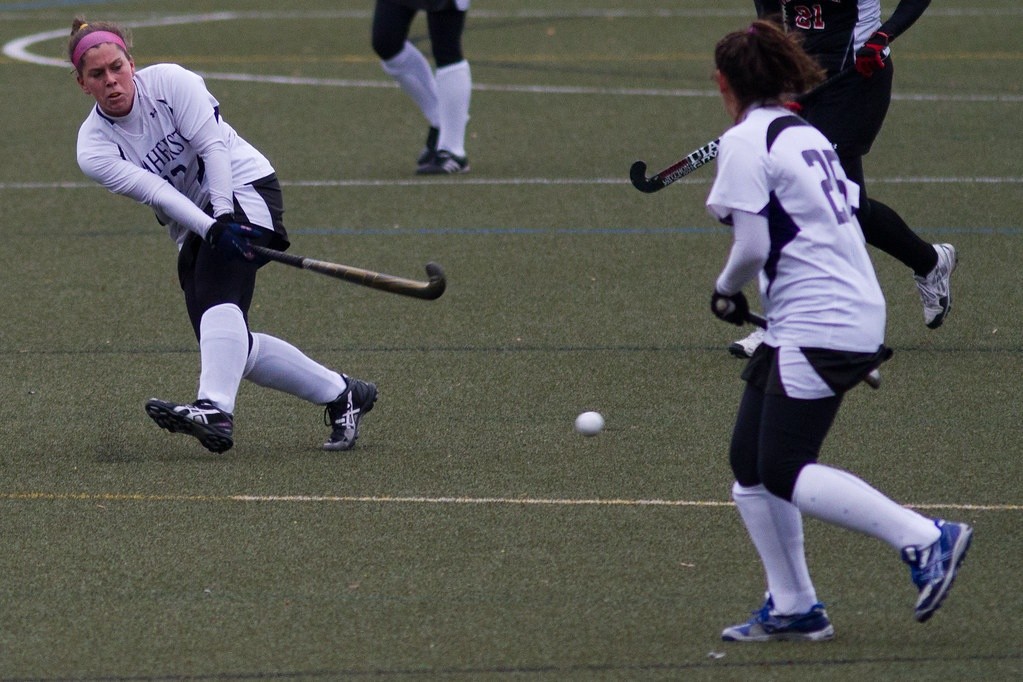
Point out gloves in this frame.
[711,290,749,327]
[209,213,239,262]
[854,31,895,77]
[207,222,263,263]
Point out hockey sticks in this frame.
[716,296,879,389]
[232,240,448,301]
[629,60,877,195]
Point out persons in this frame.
[710,19,975,642]
[371,0,472,174]
[61,13,379,455]
[729,0,958,390]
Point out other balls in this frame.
[572,411,604,436]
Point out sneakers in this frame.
[900,519,974,623]
[721,591,835,643]
[913,243,960,328]
[145,395,235,454]
[322,369,378,453]
[727,327,767,358]
[415,150,470,176]
[417,113,471,166]
